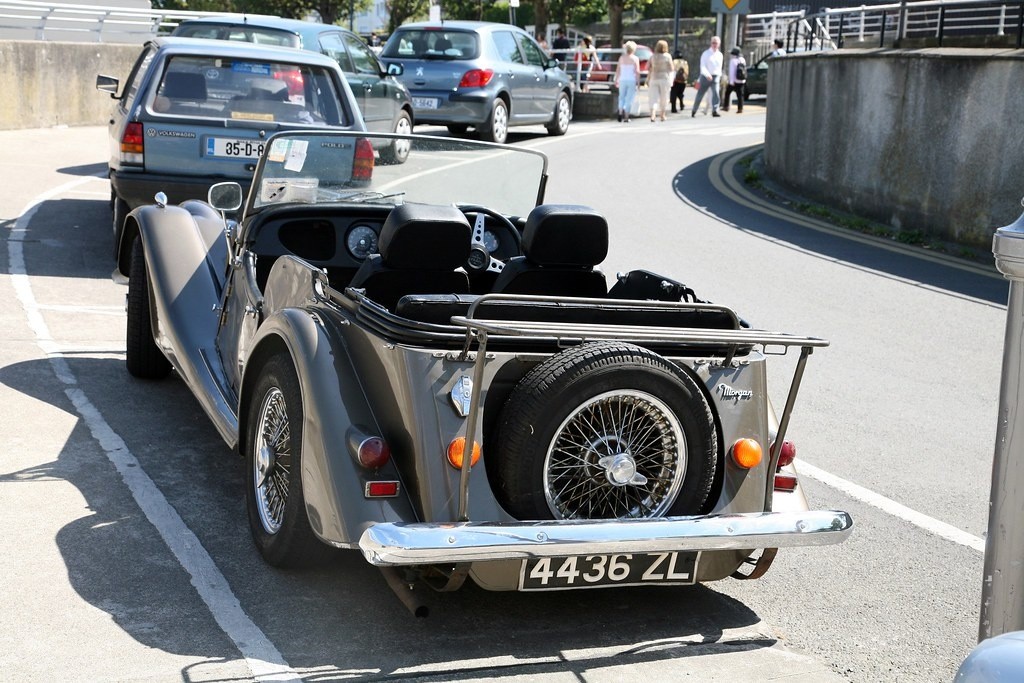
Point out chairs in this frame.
[410,39,429,57]
[433,40,452,57]
[162,72,208,109]
[347,203,473,326]
[480,204,610,323]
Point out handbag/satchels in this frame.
[575,52,588,67]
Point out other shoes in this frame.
[660,117,666,122]
[691,113,695,117]
[672,110,677,113]
[650,117,656,122]
[712,113,720,118]
[680,107,684,110]
[736,111,742,114]
[720,108,729,112]
[618,115,623,122]
[624,119,628,122]
[701,111,707,115]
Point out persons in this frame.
[552,27,570,71]
[645,40,674,122]
[770,37,786,57]
[691,36,724,118]
[720,46,748,113]
[370,32,380,46]
[574,36,602,93]
[536,31,548,49]
[613,40,640,123]
[669,50,689,113]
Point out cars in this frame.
[95,36,374,261]
[743,48,832,101]
[170,19,416,164]
[589,45,656,85]
[111,128,855,618]
[376,19,575,144]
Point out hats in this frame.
[674,50,684,58]
[731,49,740,55]
[586,36,592,42]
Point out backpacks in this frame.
[675,67,685,82]
[736,63,747,80]
